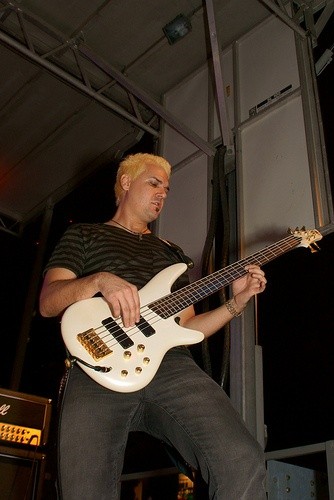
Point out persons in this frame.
[39,152,267,500]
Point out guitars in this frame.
[61,225,323,393]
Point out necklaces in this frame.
[111,218,148,240]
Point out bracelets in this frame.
[225,301,242,317]
